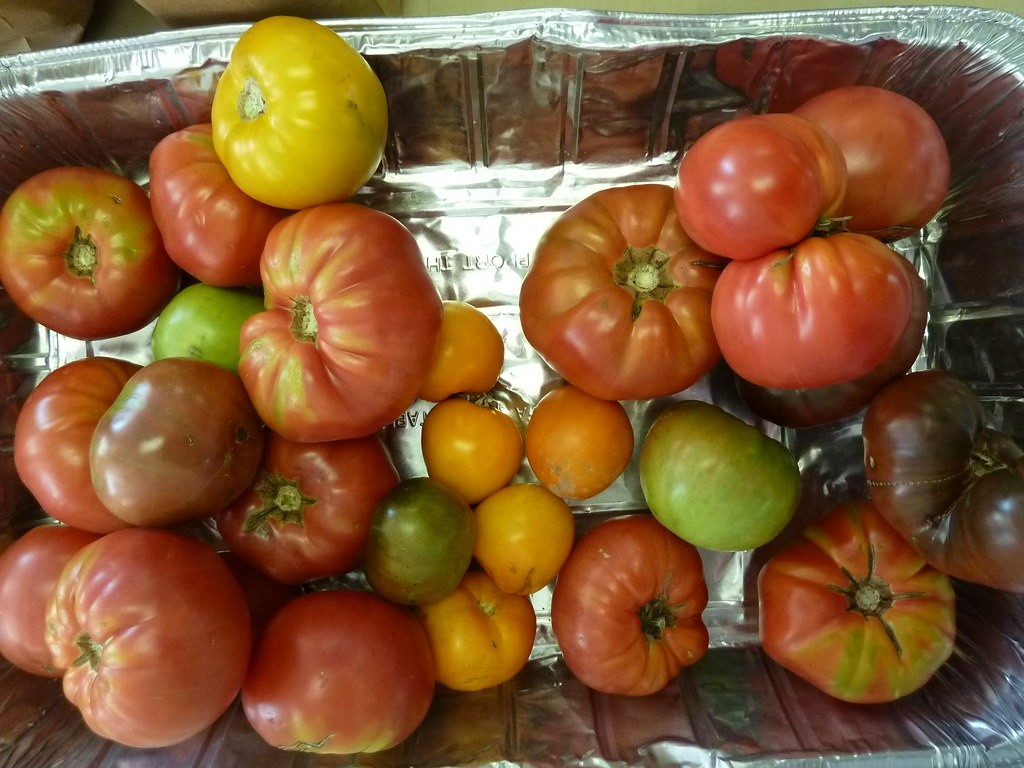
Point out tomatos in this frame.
[521,88,1024,696]
[0,13,441,755]
[368,300,635,690]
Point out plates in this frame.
[0,2,1022,768]
